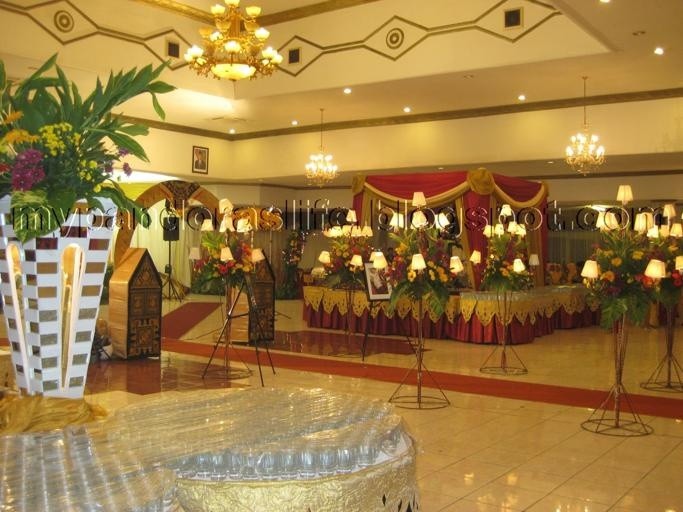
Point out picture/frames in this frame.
[364,260,392,301]
[192,145,209,173]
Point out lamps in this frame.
[200,219,214,232]
[568,132,607,174]
[582,259,598,278]
[411,210,429,229]
[659,223,669,238]
[434,212,450,231]
[219,215,234,233]
[342,224,352,239]
[346,209,357,224]
[663,204,676,218]
[508,221,517,234]
[645,212,656,228]
[517,225,527,236]
[596,211,613,230]
[674,256,683,270]
[318,250,331,263]
[449,255,463,272]
[305,153,338,188]
[514,258,526,272]
[495,222,504,237]
[634,213,648,232]
[183,0,286,79]
[412,190,427,207]
[500,204,513,217]
[670,223,683,239]
[470,250,481,265]
[350,255,362,267]
[647,226,660,241]
[250,248,266,263]
[219,199,233,213]
[220,246,235,261]
[389,212,405,227]
[412,254,426,269]
[237,217,251,233]
[351,225,362,237]
[644,259,667,279]
[529,253,540,266]
[362,226,373,237]
[485,225,495,238]
[373,254,388,268]
[616,184,633,202]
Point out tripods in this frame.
[162,243,181,302]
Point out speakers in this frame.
[164,217,178,241]
